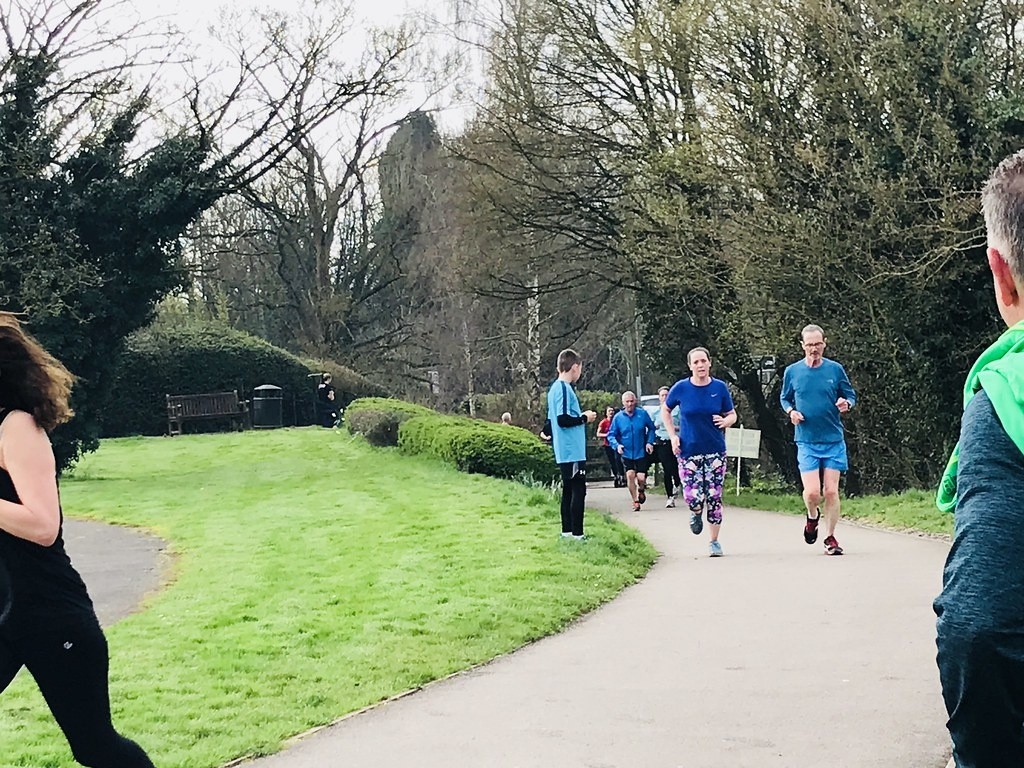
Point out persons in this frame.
[318,373,336,429]
[539,415,553,447]
[548,349,596,540]
[0,313,154,768]
[933,147,1024,768]
[780,325,856,556]
[661,347,737,557]
[596,406,634,489]
[500,412,512,427]
[649,386,683,508]
[606,391,655,511]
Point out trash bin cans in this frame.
[252,384,283,428]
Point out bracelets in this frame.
[788,408,795,415]
[847,401,852,410]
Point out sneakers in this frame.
[666,496,674,508]
[804,506,820,544]
[824,536,842,555]
[633,501,640,511]
[638,488,645,504]
[672,487,679,499]
[709,540,724,557]
[690,512,703,534]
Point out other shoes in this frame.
[561,532,595,544]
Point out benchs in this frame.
[166,390,250,437]
